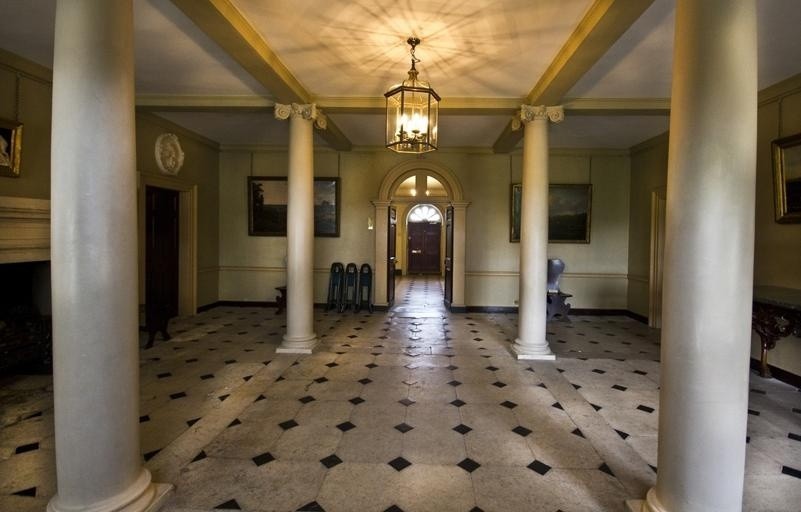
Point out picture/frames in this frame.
[1,116,24,180]
[772,134,801,226]
[248,177,340,236]
[511,183,593,244]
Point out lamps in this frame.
[384,38,444,154]
